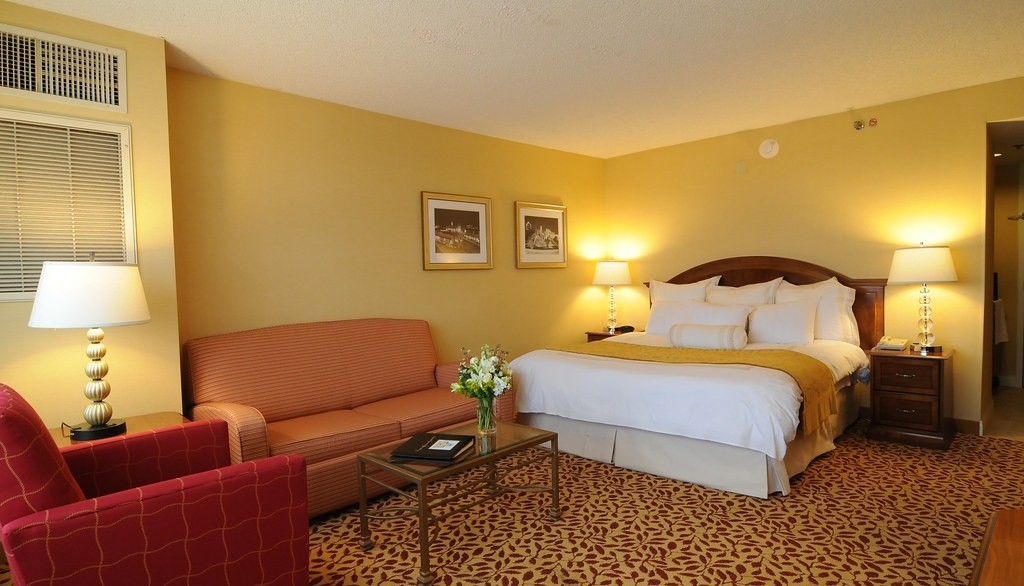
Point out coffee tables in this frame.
[357,418,562,585]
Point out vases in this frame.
[476,399,497,456]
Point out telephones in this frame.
[876,335,908,351]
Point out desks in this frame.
[969,509,1024,586]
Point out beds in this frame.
[508,256,889,500]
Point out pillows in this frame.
[682,298,755,329]
[670,324,747,350]
[647,302,685,335]
[748,294,822,344]
[705,277,784,307]
[775,276,860,347]
[650,275,721,303]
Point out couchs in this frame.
[183,318,519,519]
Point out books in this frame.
[389,432,476,464]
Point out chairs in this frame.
[1,383,311,586]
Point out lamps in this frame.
[886,242,959,347]
[28,251,152,440]
[593,258,633,334]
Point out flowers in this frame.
[451,343,513,452]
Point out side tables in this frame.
[49,412,192,448]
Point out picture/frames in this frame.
[514,201,568,269]
[420,191,494,270]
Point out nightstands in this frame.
[866,348,956,451]
[585,330,645,342]
